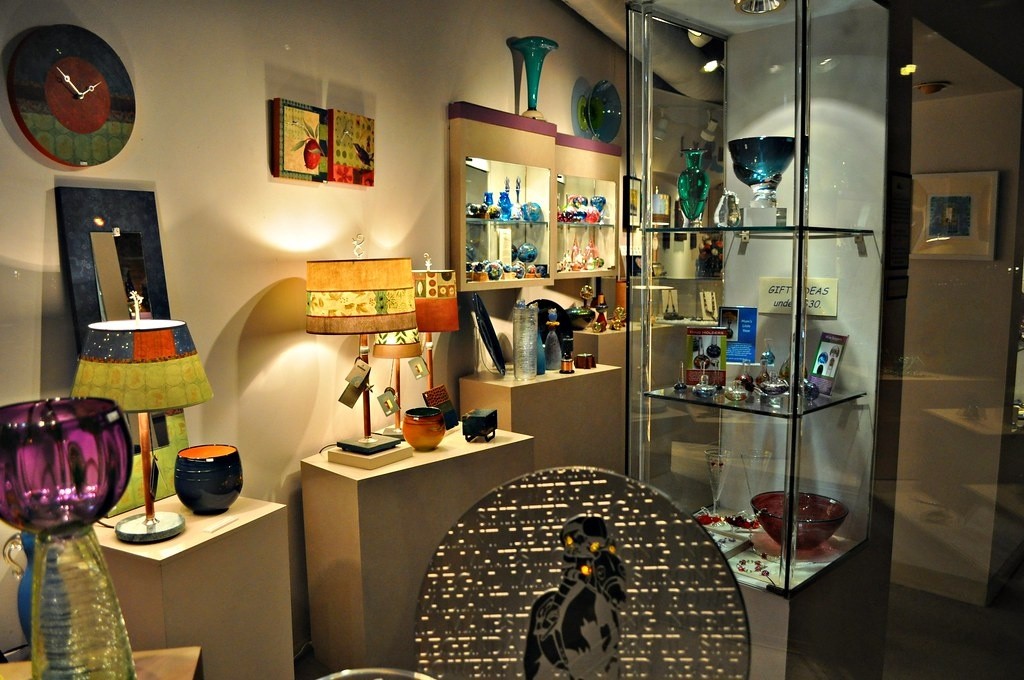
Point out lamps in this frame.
[654,110,668,141]
[913,83,950,94]
[308,233,461,470]
[71,290,213,544]
[700,110,718,141]
[688,30,713,48]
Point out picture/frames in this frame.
[910,170,1000,263]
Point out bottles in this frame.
[616,281,626,326]
[675,361,722,398]
[513,299,562,380]
[694,336,721,368]
[736,339,807,395]
[0,397,138,680]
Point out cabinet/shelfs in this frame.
[620,0,913,680]
[447,99,688,480]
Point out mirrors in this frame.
[642,14,727,514]
[53,185,172,356]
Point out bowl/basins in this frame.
[751,491,849,550]
[584,80,621,143]
[678,148,710,228]
[403,407,445,451]
[174,444,243,516]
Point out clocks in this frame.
[5,21,136,167]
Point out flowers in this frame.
[696,234,725,271]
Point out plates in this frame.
[526,299,573,357]
[473,293,506,376]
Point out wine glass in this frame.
[704,448,732,517]
[740,449,773,522]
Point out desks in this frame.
[301,421,538,670]
[0,645,203,679]
[91,490,296,679]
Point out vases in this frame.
[676,148,709,228]
[403,407,443,453]
[173,444,244,515]
[507,37,559,121]
[1,387,139,679]
